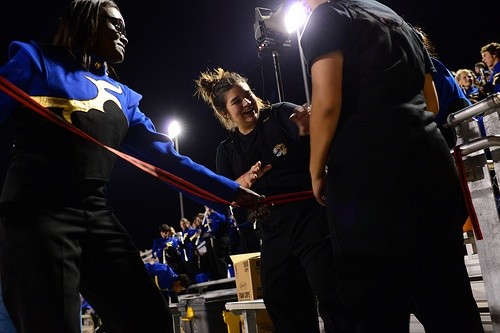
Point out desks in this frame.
[225,299,266,333]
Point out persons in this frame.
[413,27,472,149]
[193,67,356,333]
[79,205,261,333]
[450,42,500,160]
[300,0,486,333]
[0,0,274,332]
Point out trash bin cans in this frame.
[169,277,251,333]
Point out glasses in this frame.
[98,13,126,37]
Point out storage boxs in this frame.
[229,252,264,302]
